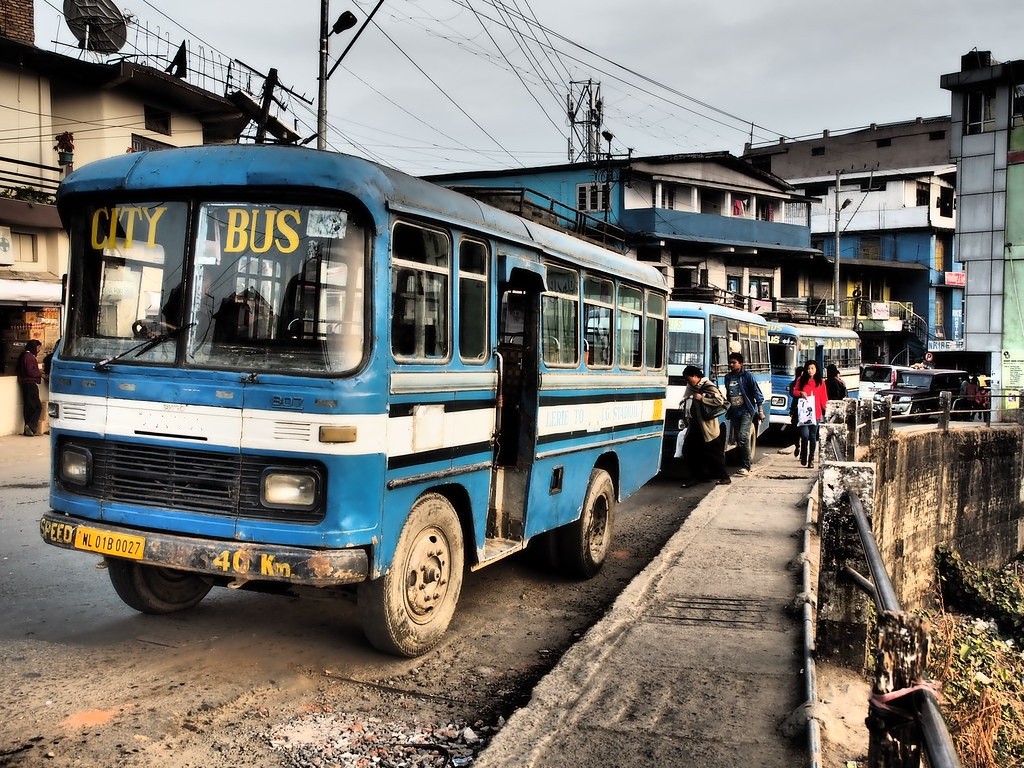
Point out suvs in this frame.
[872,369,970,422]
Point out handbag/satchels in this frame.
[698,383,731,420]
[797,395,816,426]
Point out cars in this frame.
[858,363,918,417]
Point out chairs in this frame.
[333,321,364,337]
[509,332,561,364]
[546,335,589,366]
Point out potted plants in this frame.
[53,130,75,162]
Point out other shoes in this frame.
[794,446,799,458]
[34,431,43,437]
[738,466,748,475]
[25,425,33,436]
[682,479,697,488]
[716,479,732,484]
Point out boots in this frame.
[809,455,814,468]
[800,449,807,466]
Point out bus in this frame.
[38,142,671,659]
[765,320,861,438]
[583,300,771,464]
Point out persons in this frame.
[43,339,60,377]
[725,353,765,476]
[825,365,848,399]
[789,367,804,458]
[140,268,221,343]
[678,366,731,488]
[16,340,43,436]
[793,360,828,468]
[960,377,990,421]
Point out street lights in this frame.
[317,0,358,150]
[833,198,859,320]
[602,130,614,244]
